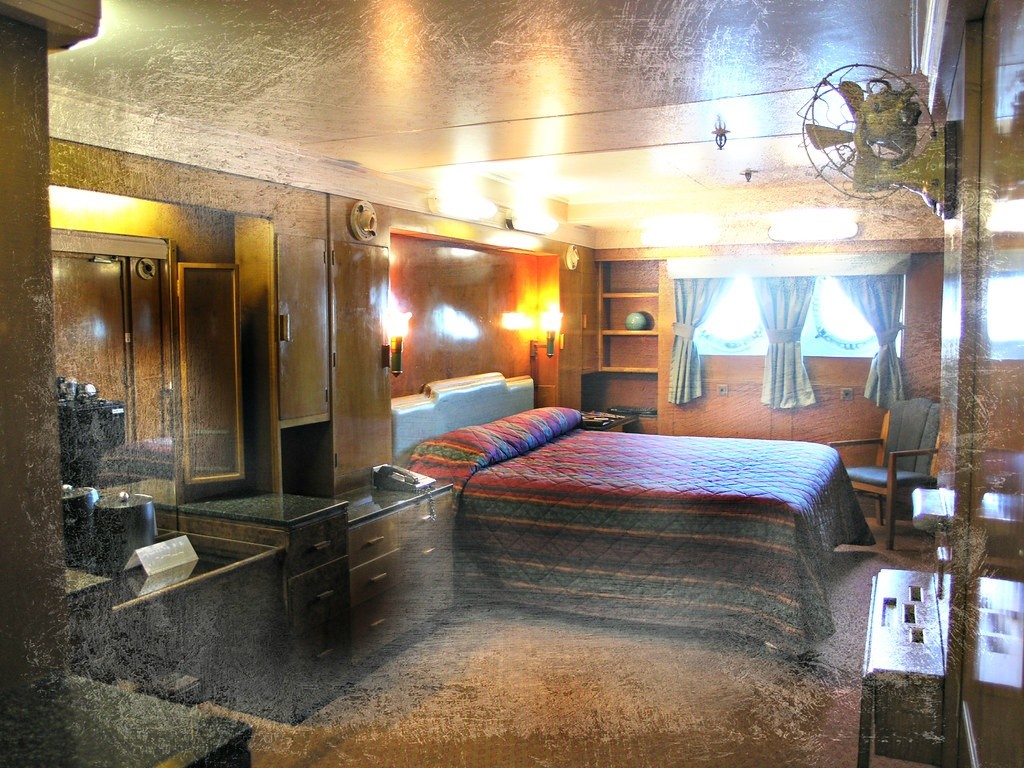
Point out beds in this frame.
[390,373,878,664]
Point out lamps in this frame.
[381,311,414,377]
[529,313,564,360]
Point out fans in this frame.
[795,62,958,219]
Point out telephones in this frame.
[371,463,437,491]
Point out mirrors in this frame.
[47,183,273,519]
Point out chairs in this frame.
[827,397,941,548]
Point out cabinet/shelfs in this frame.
[856,567,944,768]
[275,233,333,420]
[599,261,656,374]
[347,489,452,678]
[330,237,394,490]
[287,511,352,718]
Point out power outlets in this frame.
[839,388,854,402]
[718,385,728,397]
